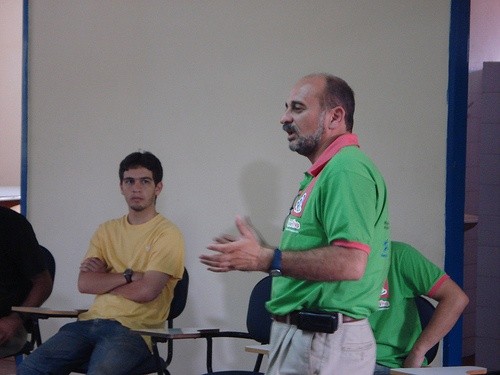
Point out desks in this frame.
[12,306,88,349]
[389,365,487,375]
[130,329,250,375]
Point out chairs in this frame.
[203,276,270,375]
[416,296,439,364]
[69,266,189,375]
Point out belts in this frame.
[272,309,361,326]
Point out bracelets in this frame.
[123,268,134,283]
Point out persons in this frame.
[368,241,468,375]
[0,206,53,357]
[200,73,391,375]
[16,151,183,375]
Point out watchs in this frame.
[267,249,282,277]
